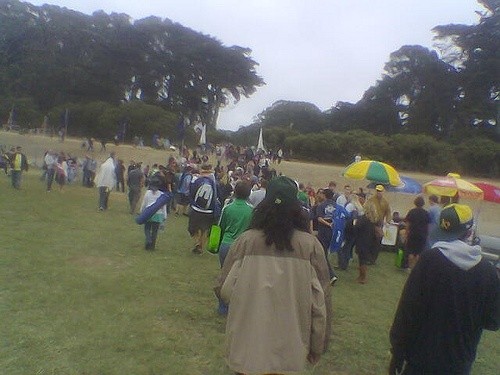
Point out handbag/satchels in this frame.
[395,248,403,268]
[207,224,222,254]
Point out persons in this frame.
[0,120,448,312]
[214,177,333,375]
[217,181,256,313]
[388,204,500,375]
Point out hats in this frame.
[375,184,384,192]
[265,175,299,205]
[435,204,473,241]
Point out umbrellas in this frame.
[367,176,422,193]
[423,173,484,201]
[342,160,403,185]
[470,183,500,242]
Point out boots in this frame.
[355,264,368,282]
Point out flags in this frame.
[199,124,207,144]
[257,132,264,150]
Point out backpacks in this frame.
[194,182,214,211]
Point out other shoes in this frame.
[98,207,104,211]
[145,241,155,250]
[330,276,337,286]
[192,244,201,254]
